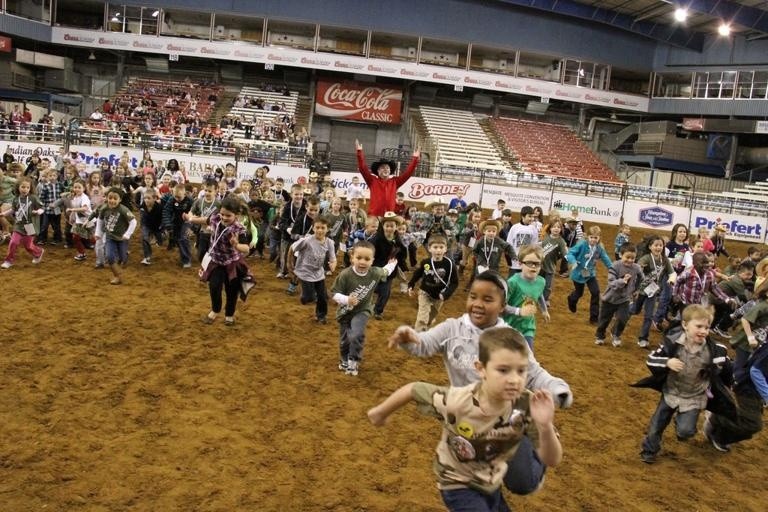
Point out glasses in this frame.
[520,261,544,267]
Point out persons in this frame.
[366,327,564,512]
[0,71,312,165]
[328,199,768,465]
[355,138,422,216]
[0,143,366,326]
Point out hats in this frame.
[372,158,396,174]
[714,225,726,232]
[446,209,459,217]
[566,217,580,223]
[309,172,320,178]
[479,219,502,234]
[378,212,404,226]
[424,196,448,209]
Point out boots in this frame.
[458,264,465,281]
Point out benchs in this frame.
[86,75,299,150]
[722,177,768,202]
[418,105,628,194]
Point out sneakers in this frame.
[702,429,728,452]
[1,262,14,268]
[183,262,191,268]
[37,240,73,249]
[545,300,550,307]
[568,295,576,313]
[710,325,732,339]
[595,336,604,345]
[640,442,656,463]
[637,340,649,347]
[590,318,600,326]
[560,273,570,278]
[318,316,326,324]
[141,257,151,264]
[32,248,44,264]
[401,288,408,294]
[611,333,621,347]
[652,320,662,332]
[326,264,345,276]
[375,312,383,320]
[74,253,127,284]
[204,315,234,326]
[270,253,296,295]
[338,360,359,376]
